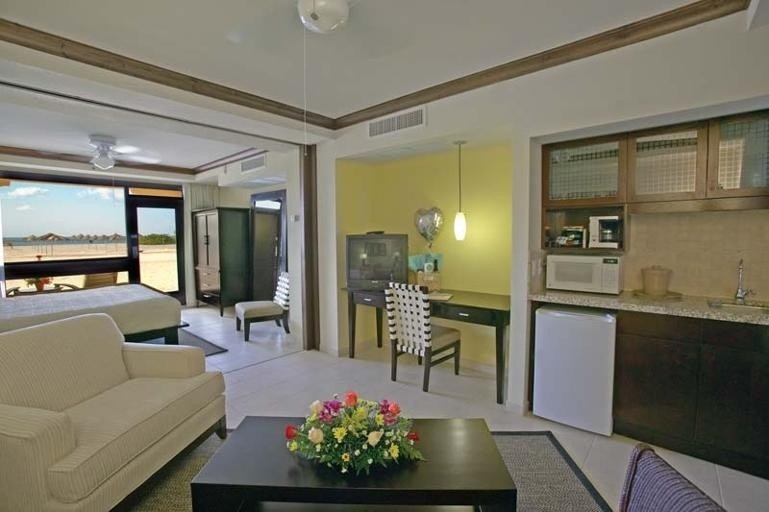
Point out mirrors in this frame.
[250,189,287,300]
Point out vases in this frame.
[36,284,44,291]
[347,468,377,484]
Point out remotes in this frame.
[366,231,385,234]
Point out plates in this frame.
[630,288,683,300]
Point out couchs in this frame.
[0,312,226,512]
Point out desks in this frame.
[340,288,510,404]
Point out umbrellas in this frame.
[24,232,125,253]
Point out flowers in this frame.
[24,277,52,287]
[284,392,432,476]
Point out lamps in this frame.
[454,140,466,241]
[297,0,349,34]
[88,134,117,170]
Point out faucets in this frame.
[735,258,757,299]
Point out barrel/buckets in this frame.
[642,266,673,298]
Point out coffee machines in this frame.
[588,214,624,250]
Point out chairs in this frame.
[235,272,289,341]
[384,282,460,391]
[54,271,117,292]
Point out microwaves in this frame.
[545,253,626,295]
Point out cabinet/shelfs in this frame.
[614,311,768,480]
[542,106,769,255]
[191,207,250,316]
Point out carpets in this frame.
[144,328,229,357]
[108,429,613,512]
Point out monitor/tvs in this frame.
[345,234,408,292]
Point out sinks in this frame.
[708,299,768,310]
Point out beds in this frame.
[1,283,190,344]
